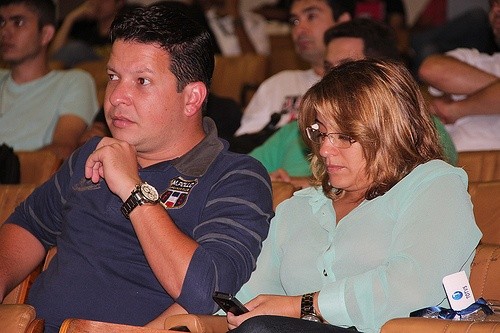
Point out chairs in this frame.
[0,38,500,333]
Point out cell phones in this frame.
[212,292,250,316]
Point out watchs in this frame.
[121,181,160,220]
[300,292,323,323]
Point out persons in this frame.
[47,0,127,70]
[247,18,460,192]
[408,0,500,153]
[214,57,483,333]
[204,0,490,57]
[229,0,358,155]
[0,0,101,185]
[0,1,275,332]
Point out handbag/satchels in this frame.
[410,295,494,321]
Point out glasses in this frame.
[306,122,357,148]
